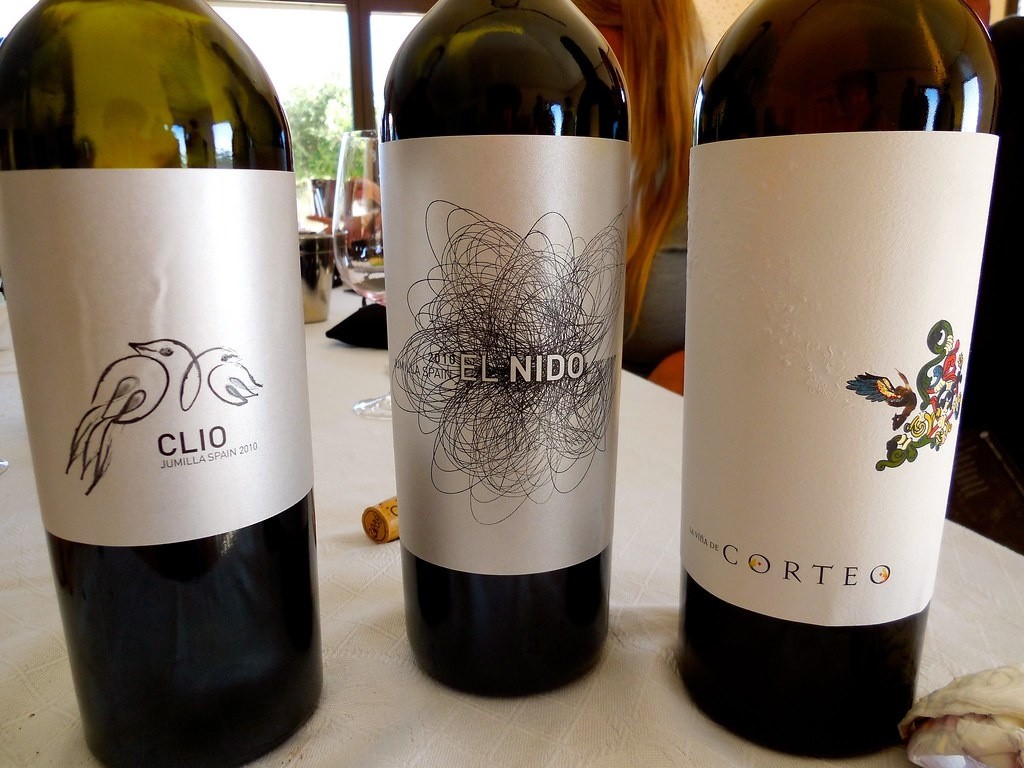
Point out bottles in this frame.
[0,0,322,768]
[378,0,629,700]
[677,0,1000,761]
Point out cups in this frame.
[312,179,355,218]
[298,230,333,324]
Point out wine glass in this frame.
[332,130,391,421]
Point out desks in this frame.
[0,289,1024,768]
